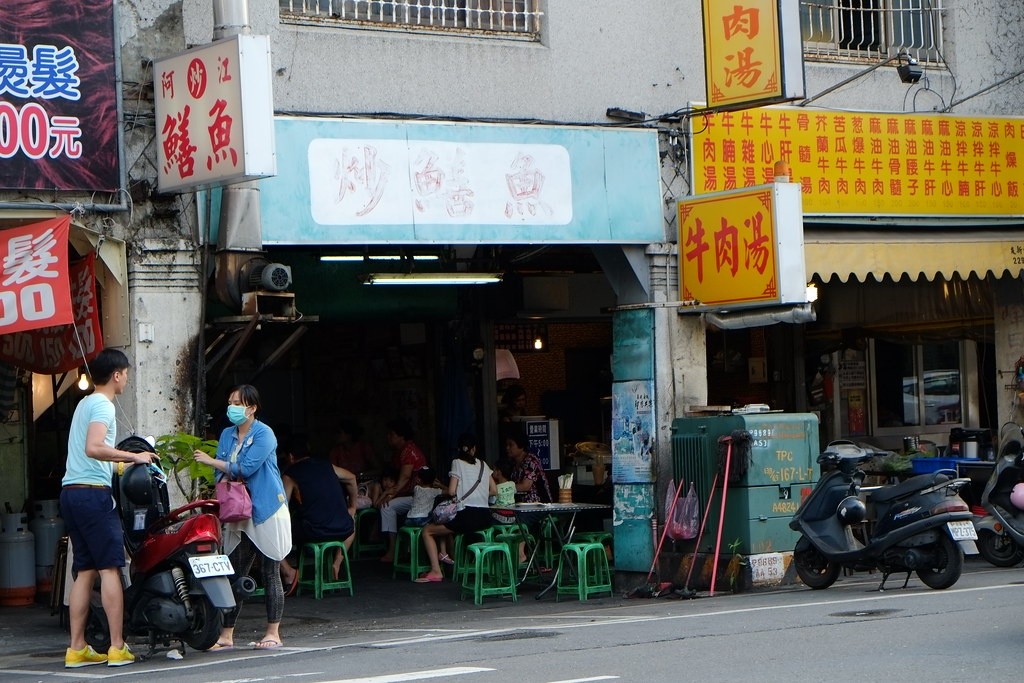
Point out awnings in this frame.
[805,225,1024,283]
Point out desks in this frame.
[466,502,615,598]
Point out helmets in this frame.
[836,495,867,526]
[122,463,155,506]
[1010,483,1024,511]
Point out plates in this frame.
[516,502,543,507]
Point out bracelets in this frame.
[118,462,124,476]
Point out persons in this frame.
[367,420,454,565]
[413,432,497,583]
[487,385,551,563]
[192,383,293,651]
[281,438,360,596]
[60,347,161,668]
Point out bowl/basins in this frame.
[903,435,920,453]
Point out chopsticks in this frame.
[557,473,574,489]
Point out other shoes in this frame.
[380,554,392,562]
[438,552,454,564]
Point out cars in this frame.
[902,369,962,426]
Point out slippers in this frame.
[284,568,299,596]
[531,567,552,573]
[206,642,234,652]
[417,573,447,583]
[254,640,283,650]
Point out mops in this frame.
[709,429,755,601]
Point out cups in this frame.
[559,489,572,503]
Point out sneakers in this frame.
[65,644,108,667]
[107,642,135,666]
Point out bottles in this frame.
[987,447,995,462]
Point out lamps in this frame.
[800,49,922,107]
[365,268,501,287]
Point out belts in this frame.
[63,484,109,489]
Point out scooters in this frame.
[64,435,259,665]
[788,439,980,592]
[974,422,1024,569]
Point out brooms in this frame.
[671,473,718,600]
[636,477,684,600]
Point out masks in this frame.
[227,405,253,427]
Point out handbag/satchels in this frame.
[216,462,252,522]
[347,482,372,510]
[433,497,457,524]
[664,479,700,540]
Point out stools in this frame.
[234,510,614,602]
